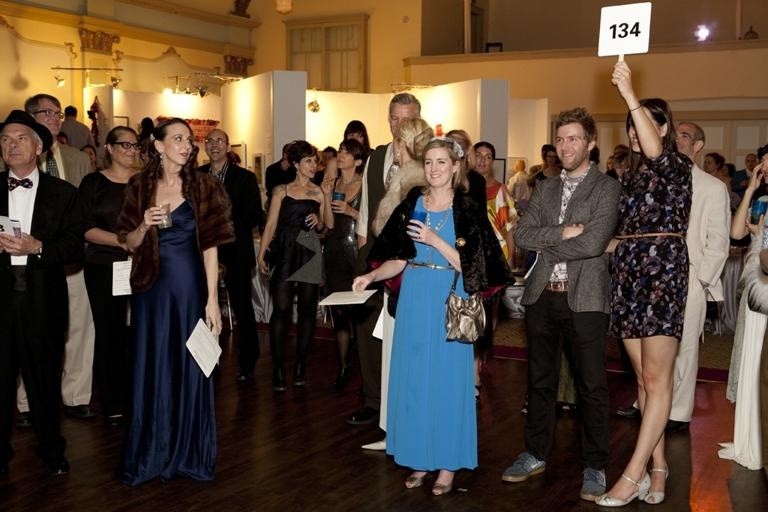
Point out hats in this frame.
[0,108,53,155]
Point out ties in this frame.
[46,147,59,180]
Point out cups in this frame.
[332,192,345,216]
[409,210,426,238]
[749,199,768,224]
[156,204,173,231]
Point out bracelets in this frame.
[628,102,644,114]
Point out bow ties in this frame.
[7,177,34,192]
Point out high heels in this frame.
[644,463,669,506]
[594,470,652,508]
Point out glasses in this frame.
[111,141,141,151]
[204,138,229,146]
[31,108,65,120]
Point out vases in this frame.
[500,283,527,319]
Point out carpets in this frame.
[256,311,768,387]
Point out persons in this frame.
[595,60,693,507]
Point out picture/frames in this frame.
[111,115,129,130]
[251,152,266,188]
[230,140,249,171]
[490,157,507,186]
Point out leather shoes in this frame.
[345,405,380,426]
[360,437,386,452]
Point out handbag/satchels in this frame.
[443,268,487,345]
[264,239,285,270]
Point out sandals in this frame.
[432,479,454,497]
[474,381,482,407]
[519,393,530,416]
[404,471,435,490]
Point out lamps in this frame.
[51,64,126,88]
[307,87,322,115]
[161,75,213,99]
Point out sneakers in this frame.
[15,411,33,428]
[580,465,607,502]
[64,404,97,419]
[235,365,256,383]
[501,451,547,483]
[271,367,288,393]
[328,366,352,394]
[664,420,690,435]
[615,405,641,420]
[291,363,306,388]
[46,459,69,475]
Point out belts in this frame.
[544,280,569,293]
